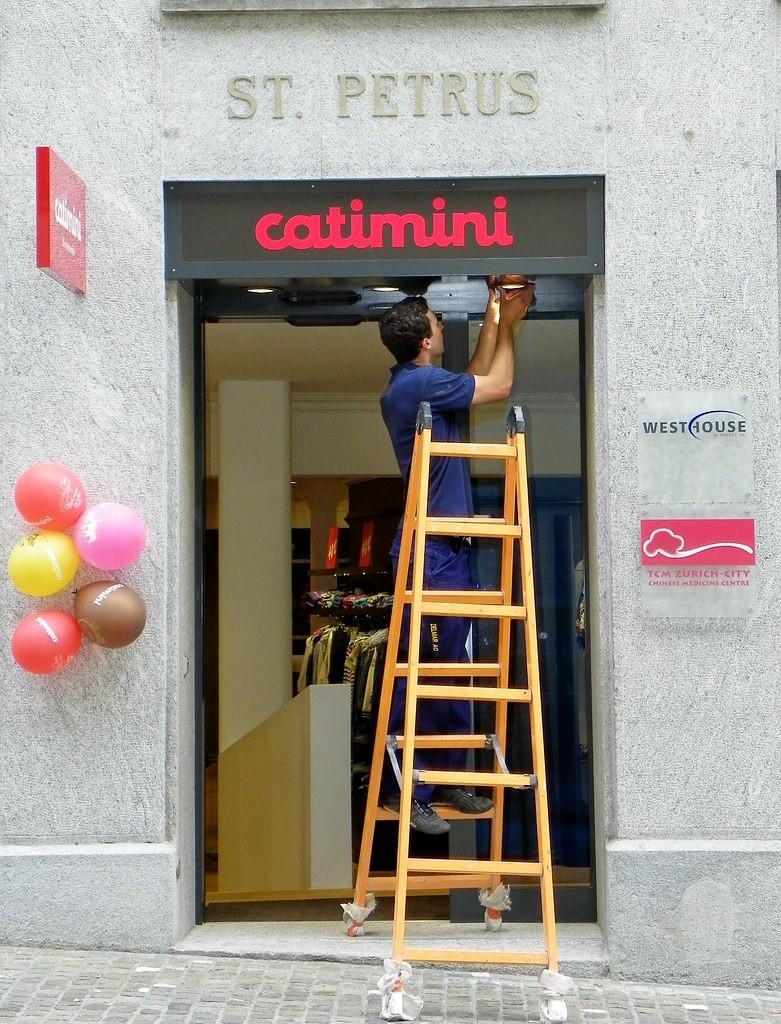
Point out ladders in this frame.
[340,400,574,1024]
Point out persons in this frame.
[377,279,536,834]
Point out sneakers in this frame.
[429,787,497,815]
[388,796,451,835]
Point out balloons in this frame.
[71,502,145,570]
[14,462,85,532]
[74,580,145,650]
[7,530,79,597]
[10,608,81,676]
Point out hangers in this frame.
[304,616,391,653]
